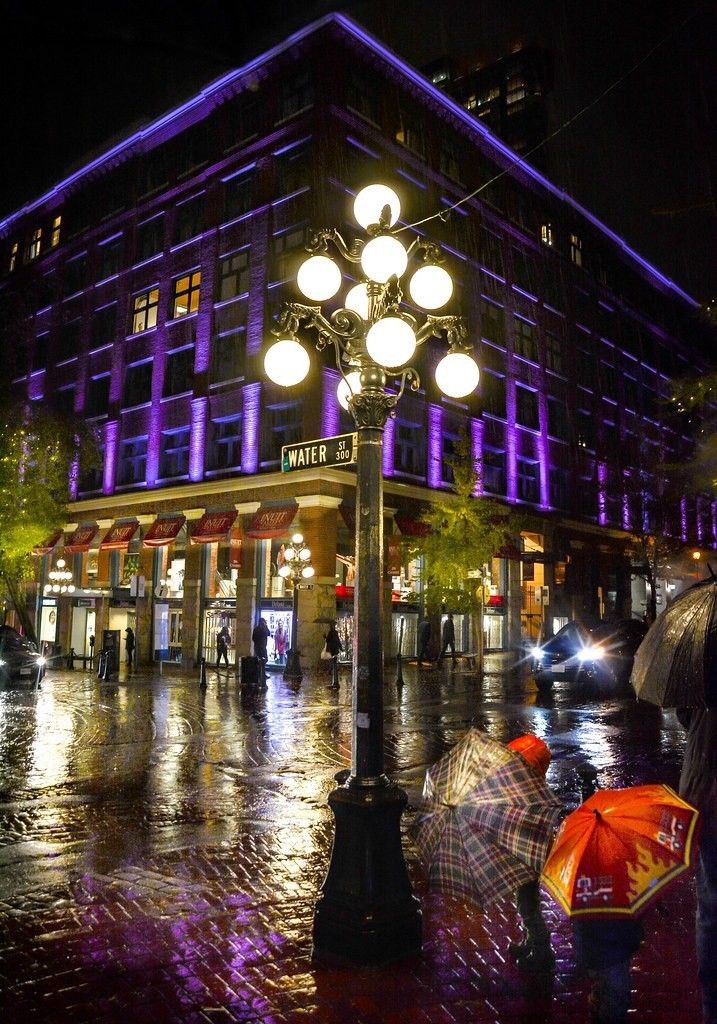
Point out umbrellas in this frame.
[629,562,717,710]
[312,617,337,634]
[405,725,566,913]
[541,785,704,924]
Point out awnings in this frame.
[100,521,139,550]
[338,504,356,537]
[486,517,522,560]
[31,533,61,554]
[64,526,99,552]
[394,515,437,549]
[247,503,299,539]
[191,510,238,543]
[142,516,186,549]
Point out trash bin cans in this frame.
[239,656,258,684]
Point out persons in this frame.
[676,708,717,1024]
[274,620,287,665]
[436,614,458,665]
[417,617,432,667]
[505,734,558,972]
[217,626,233,670]
[124,627,136,666]
[323,624,344,689]
[579,914,643,1024]
[253,618,271,679]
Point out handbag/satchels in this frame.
[320,640,332,660]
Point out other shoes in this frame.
[516,956,553,967]
[507,941,529,957]
[417,663,423,666]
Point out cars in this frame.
[531,617,649,696]
[0,624,46,691]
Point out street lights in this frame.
[277,533,315,683]
[263,182,483,968]
